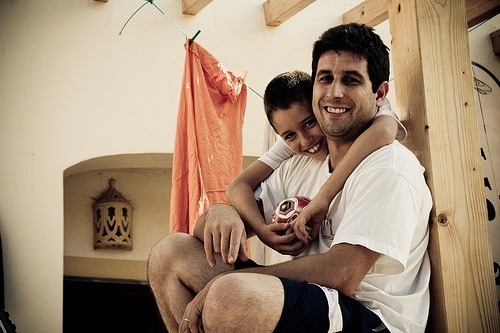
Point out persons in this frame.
[225,68,409,258]
[147,22,435,333]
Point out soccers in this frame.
[272,196,314,244]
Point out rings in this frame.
[184,318,190,322]
